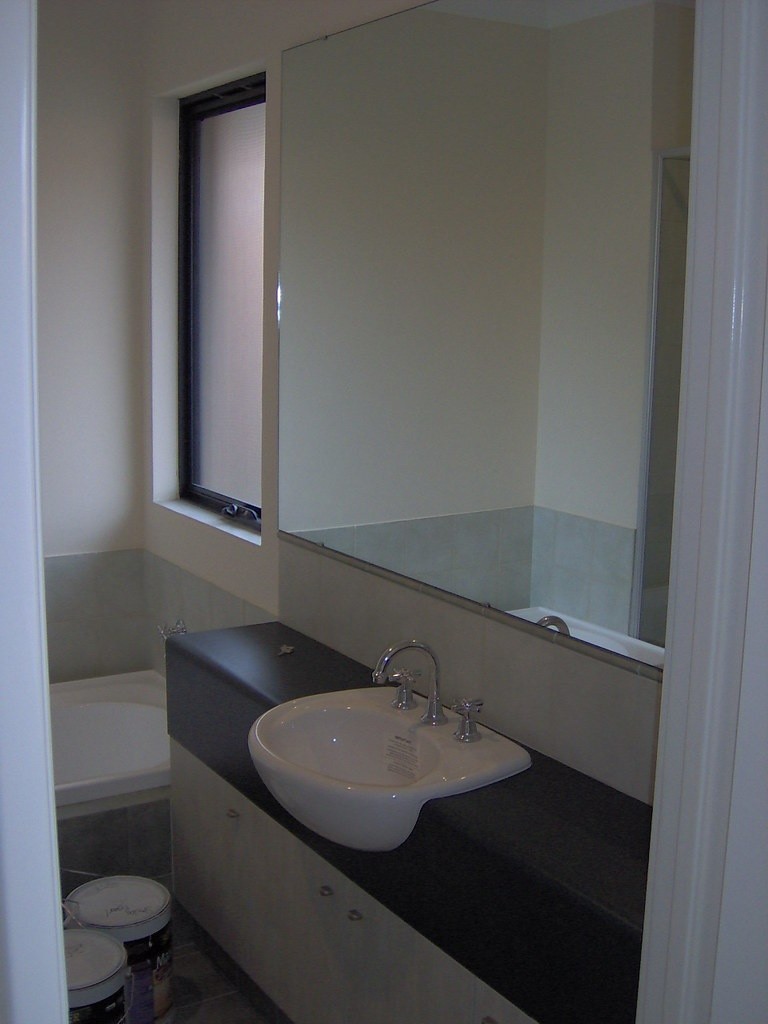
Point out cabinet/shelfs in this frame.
[168,735,536,1024]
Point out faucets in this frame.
[536,615,572,636]
[372,638,449,727]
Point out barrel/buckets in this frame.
[60,865,175,1024]
[63,928,135,1024]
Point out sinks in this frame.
[247,684,533,853]
[503,606,666,671]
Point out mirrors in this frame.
[279,0,696,676]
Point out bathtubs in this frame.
[49,668,173,822]
